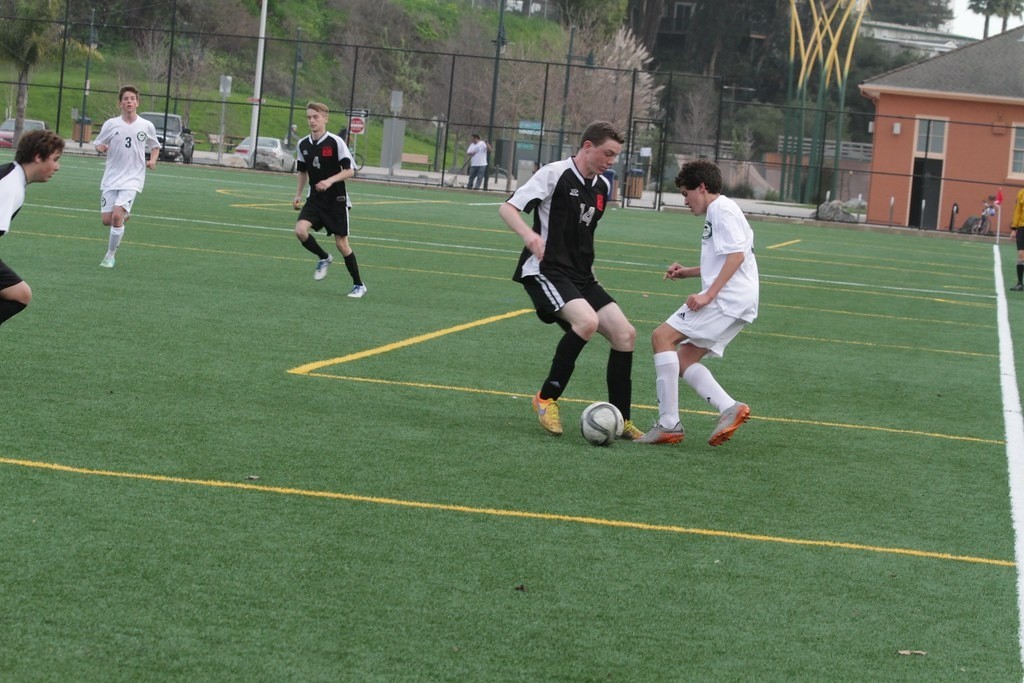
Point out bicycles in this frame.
[971,199,995,236]
[349,148,364,171]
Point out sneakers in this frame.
[632,417,687,444]
[348,280,367,298]
[314,254,333,280]
[100,255,116,268]
[708,402,750,446]
[532,391,564,435]
[622,420,646,439]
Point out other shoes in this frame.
[1010,283,1024,290]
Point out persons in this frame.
[338,127,353,145]
[293,103,367,298]
[631,160,759,445]
[467,134,492,190]
[94,85,160,268]
[498,121,644,441]
[283,124,299,144]
[1009,188,1024,291]
[0,129,65,324]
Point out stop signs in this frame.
[348,116,366,135]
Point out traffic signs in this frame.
[344,108,371,118]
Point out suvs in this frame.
[138,111,194,164]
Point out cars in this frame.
[0,118,47,147]
[234,136,296,173]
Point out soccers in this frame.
[579,402,625,447]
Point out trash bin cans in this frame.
[73,118,92,143]
[624,169,643,199]
[606,169,620,209]
